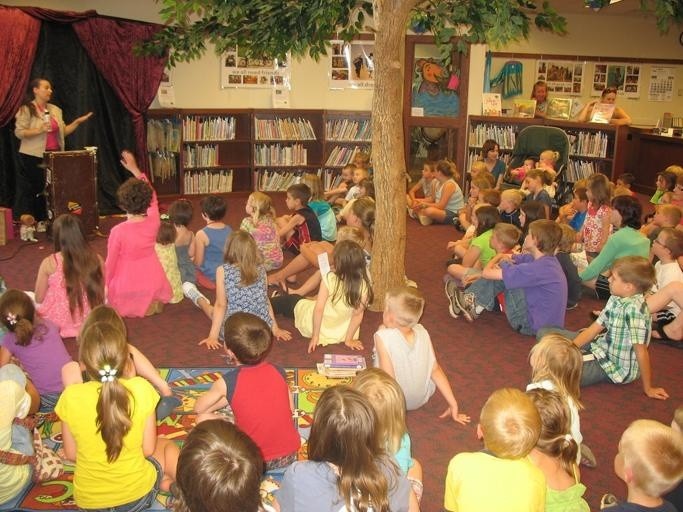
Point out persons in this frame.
[372,285,471,425]
[530,81,548,117]
[26,151,376,351]
[407,140,683,400]
[0,289,422,512]
[579,89,632,125]
[14,78,93,232]
[444,334,683,512]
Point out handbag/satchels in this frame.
[33,427,65,481]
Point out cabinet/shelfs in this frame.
[322,109,374,192]
[546,119,628,187]
[146,107,253,193]
[250,108,327,192]
[467,115,545,178]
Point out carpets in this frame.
[0,362,366,512]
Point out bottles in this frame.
[44,111,52,133]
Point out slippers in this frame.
[651,326,683,349]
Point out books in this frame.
[316,354,366,379]
[255,117,316,191]
[324,119,373,192]
[182,115,236,195]
[466,123,608,182]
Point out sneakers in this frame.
[453,288,482,322]
[37,220,47,233]
[419,215,432,225]
[444,278,461,319]
[181,281,211,309]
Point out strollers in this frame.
[497,123,577,227]
